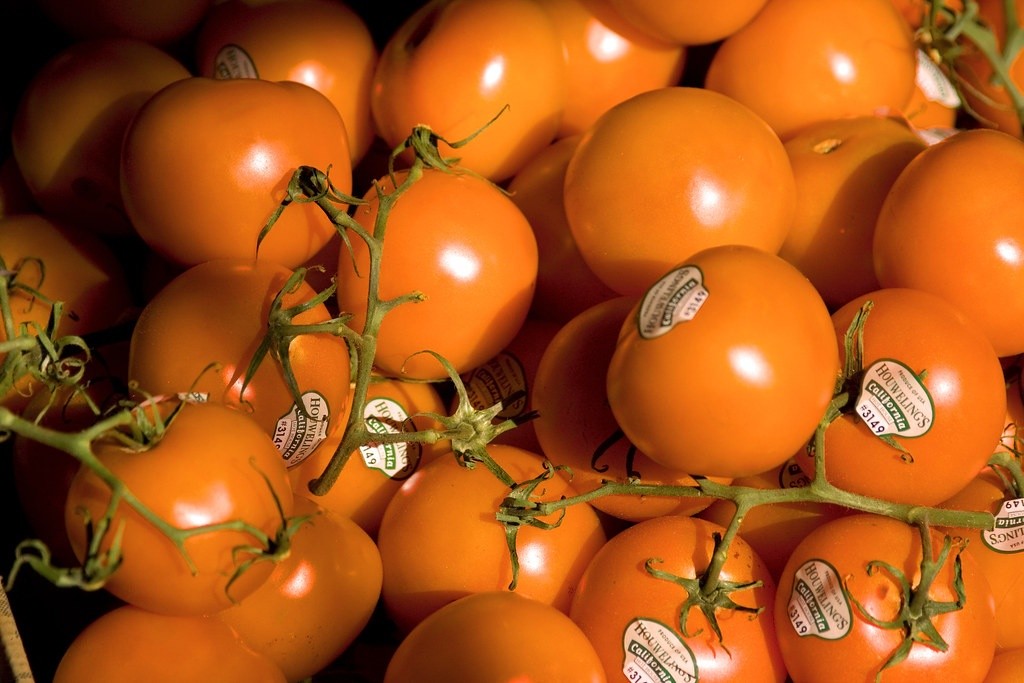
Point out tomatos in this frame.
[0,0,1024,683]
